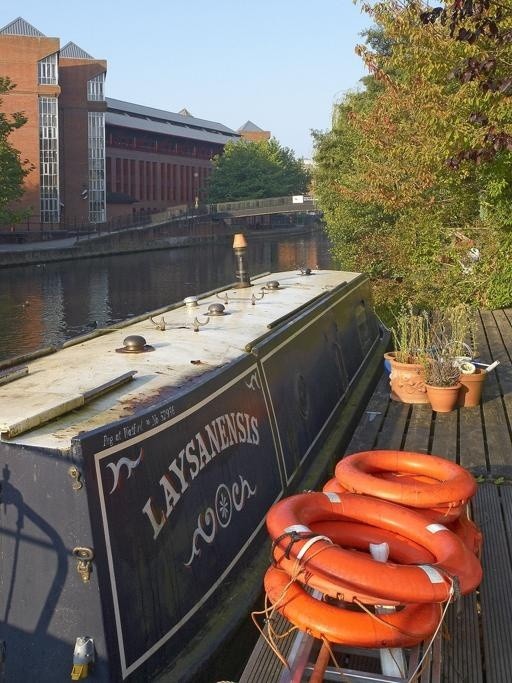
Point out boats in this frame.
[0,234,392,682]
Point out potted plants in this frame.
[422,348,463,412]
[370,298,487,412]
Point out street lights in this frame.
[194,173,199,209]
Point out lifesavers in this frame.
[335,450,478,509]
[270,518,438,606]
[324,477,467,529]
[265,490,483,605]
[439,515,482,569]
[264,560,444,648]
